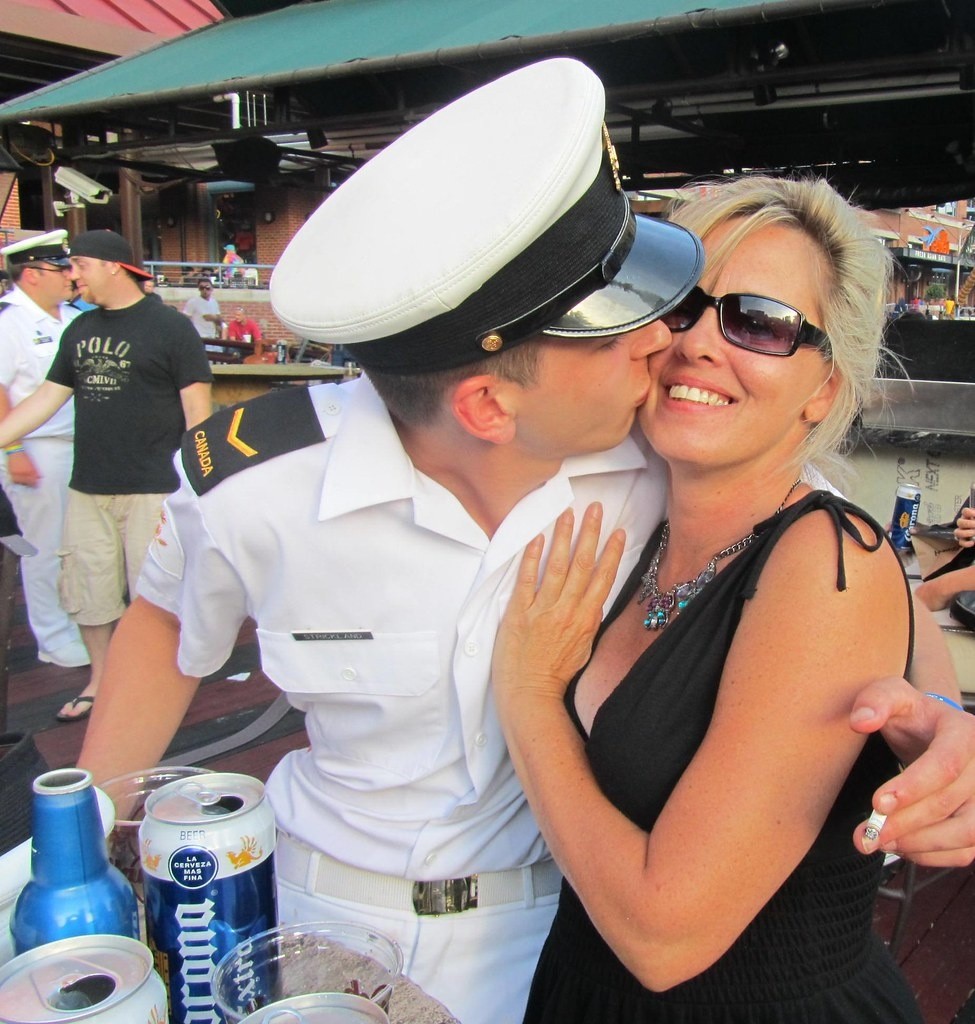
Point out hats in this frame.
[2,229,72,267]
[269,55,705,374]
[69,228,154,282]
[224,244,235,252]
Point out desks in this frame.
[208,351,363,385]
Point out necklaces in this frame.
[636,476,804,631]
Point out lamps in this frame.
[307,127,329,150]
[959,64,975,91]
[753,80,779,106]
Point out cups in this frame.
[208,919,402,1023]
[95,766,219,944]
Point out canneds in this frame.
[0,934,169,1024]
[137,772,282,1024]
[892,481,922,551]
[275,339,288,365]
[239,993,392,1024]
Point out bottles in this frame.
[9,766,142,962]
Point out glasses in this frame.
[660,287,832,358]
[29,265,72,275]
[198,284,211,290]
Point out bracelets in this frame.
[924,692,963,711]
[5,445,24,454]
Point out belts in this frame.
[274,831,563,917]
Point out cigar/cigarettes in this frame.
[864,809,887,840]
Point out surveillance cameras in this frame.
[55,166,112,204]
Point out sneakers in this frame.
[37,640,91,668]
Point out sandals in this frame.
[57,694,96,723]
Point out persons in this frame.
[142,279,164,304]
[490,180,931,1024]
[213,243,243,278]
[1,228,216,721]
[1,59,975,1024]
[0,230,96,667]
[182,276,221,365]
[67,280,100,312]
[882,492,975,610]
[182,266,223,284]
[228,307,262,344]
